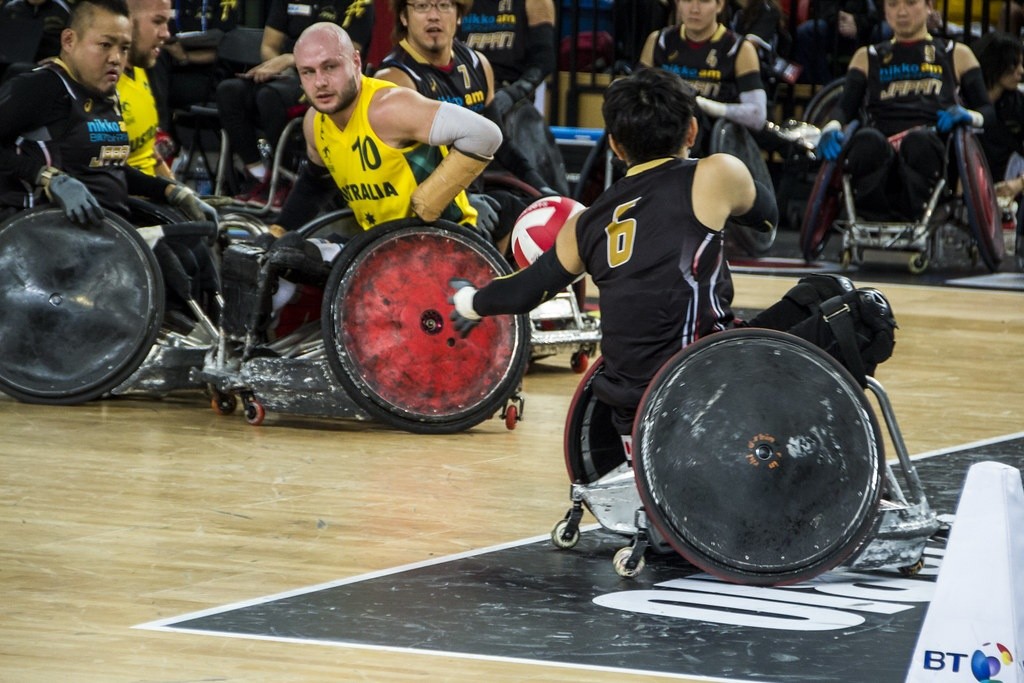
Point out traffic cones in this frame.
[902,461,1024,683]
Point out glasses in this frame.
[406,0,455,14]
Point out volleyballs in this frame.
[509,194,591,270]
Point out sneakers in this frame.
[232,174,271,206]
[246,186,292,212]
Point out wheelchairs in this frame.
[550,332,957,583]
[795,118,1015,274]
[0,37,856,435]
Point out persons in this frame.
[218,0,375,211]
[448,67,899,467]
[0,0,229,342]
[958,32,1024,207]
[373,0,528,257]
[816,0,983,216]
[639,0,767,129]
[257,31,502,316]
[716,0,895,86]
[456,0,569,197]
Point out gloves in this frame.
[936,104,973,132]
[816,125,844,160]
[493,89,514,117]
[447,277,483,338]
[167,185,220,247]
[48,172,106,231]
[465,192,501,243]
